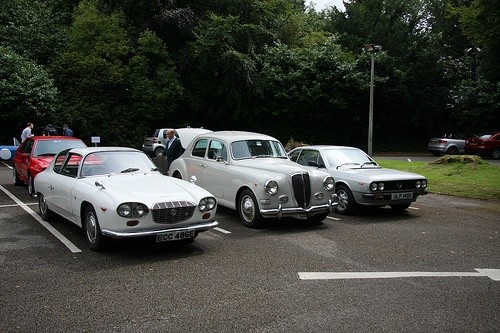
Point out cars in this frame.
[167,130,339,228]
[428,130,480,156]
[142,127,214,158]
[464,131,500,160]
[287,145,428,215]
[33,146,219,252]
[12,136,105,196]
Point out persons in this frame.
[21,123,73,144]
[159,129,182,169]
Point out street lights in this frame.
[362,43,383,157]
